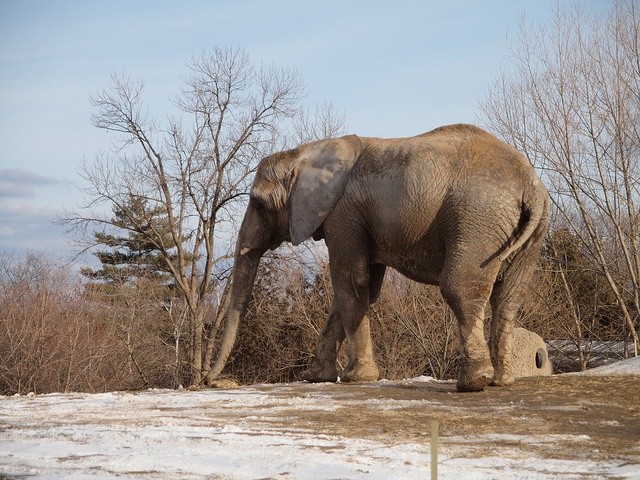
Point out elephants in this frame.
[197,123,552,392]
